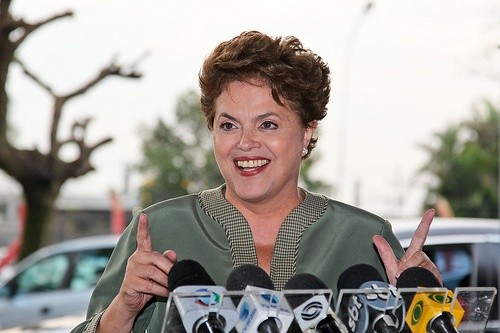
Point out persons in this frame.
[69,30,443,333]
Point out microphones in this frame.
[167,258,465,333]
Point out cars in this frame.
[0,236,121,329]
[392,217,500,333]
